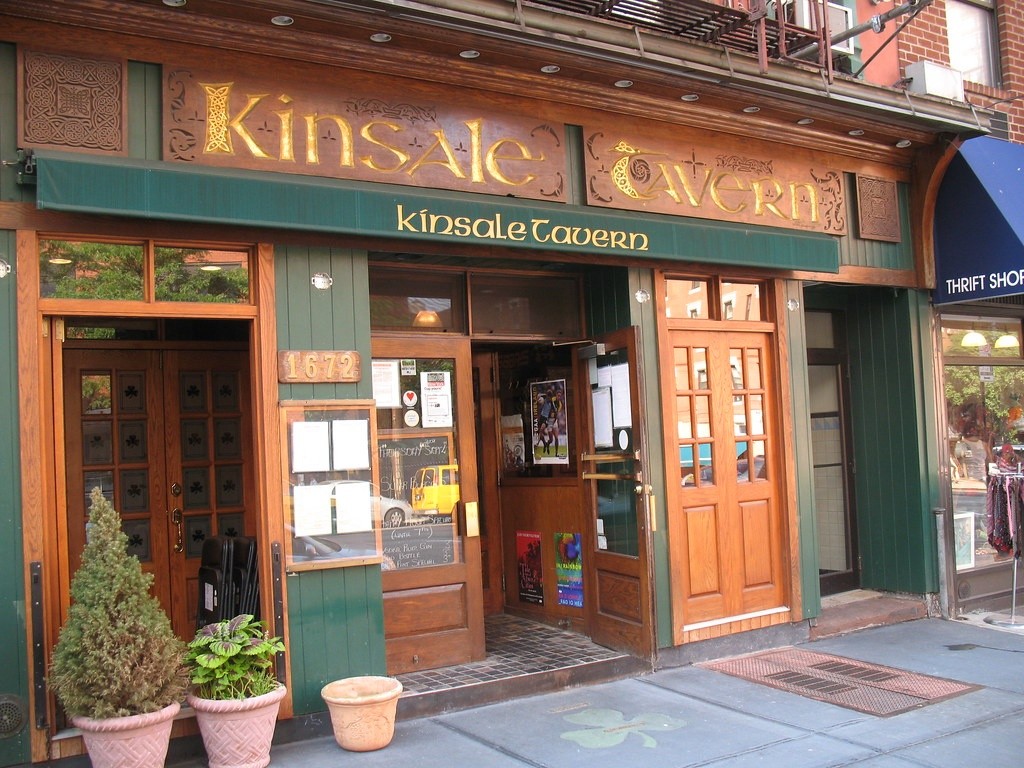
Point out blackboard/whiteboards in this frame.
[279,398,384,572]
[376,426,461,571]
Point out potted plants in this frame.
[44,488,187,768]
[187,616,287,768]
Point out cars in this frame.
[682,457,767,483]
[284,481,338,534]
[289,527,397,574]
[312,480,413,526]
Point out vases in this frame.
[322,675,403,752]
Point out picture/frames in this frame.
[520,374,573,473]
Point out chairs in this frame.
[194,537,261,634]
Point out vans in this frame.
[411,465,461,521]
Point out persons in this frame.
[953,415,998,535]
[530,388,565,457]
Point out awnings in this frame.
[930,133,1024,308]
